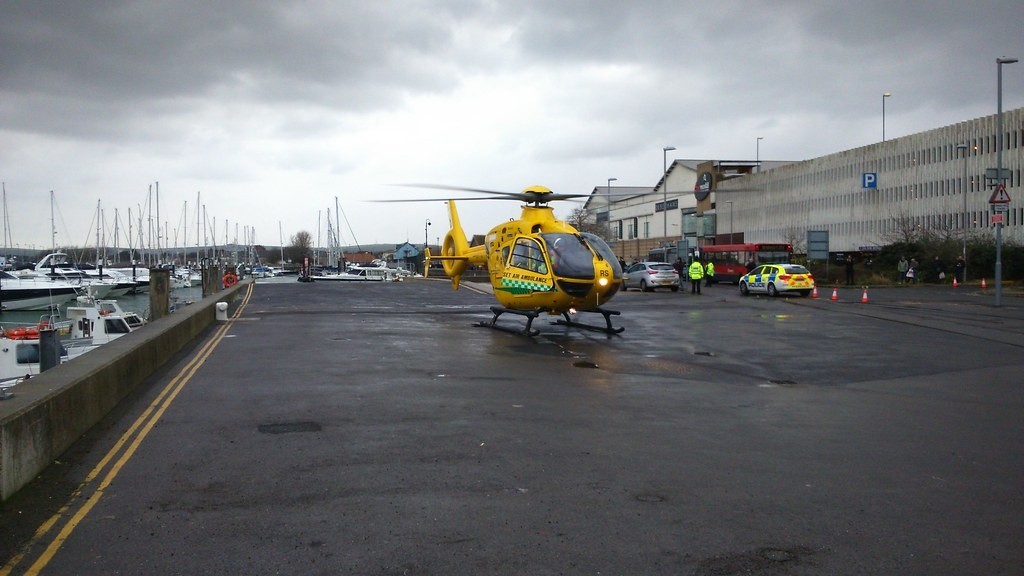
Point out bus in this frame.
[700,243,791,284]
[648,246,699,281]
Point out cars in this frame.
[739,263,815,297]
[620,261,680,292]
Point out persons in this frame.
[898,256,966,284]
[673,257,715,295]
[746,259,756,273]
[845,255,854,285]
[520,238,567,274]
[617,256,638,273]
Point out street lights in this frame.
[994,56,1019,307]
[425,218,431,248]
[607,178,617,246]
[663,146,676,262]
[882,93,891,142]
[725,200,733,245]
[756,137,764,172]
[956,143,968,283]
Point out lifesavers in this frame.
[38,322,51,329]
[99,310,110,314]
[223,274,237,287]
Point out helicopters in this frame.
[365,182,764,337]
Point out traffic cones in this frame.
[861,289,869,303]
[830,287,839,300]
[812,285,818,298]
[981,277,988,288]
[952,276,959,287]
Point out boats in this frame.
[0,280,148,392]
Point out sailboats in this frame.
[0,181,298,311]
[308,196,424,281]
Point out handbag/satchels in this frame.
[906,268,915,278]
[939,272,946,279]
